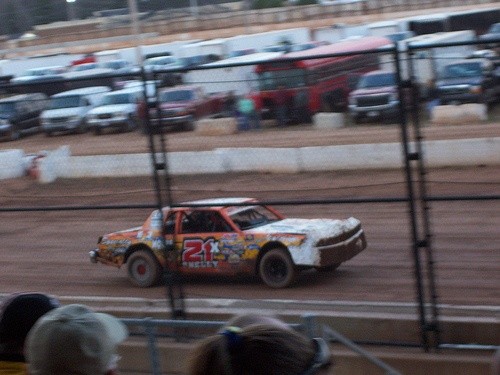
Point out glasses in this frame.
[303,336,333,374]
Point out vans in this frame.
[38,86,112,137]
[0,92,48,143]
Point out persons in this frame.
[191,310,334,374]
[0,287,128,375]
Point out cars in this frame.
[348,69,414,124]
[434,57,496,106]
[84,84,158,136]
[89,196,367,289]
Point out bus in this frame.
[247,34,395,125]
[0,7,500,98]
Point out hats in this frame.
[25,303,130,374]
[0,294,62,346]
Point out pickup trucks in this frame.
[138,84,236,135]
